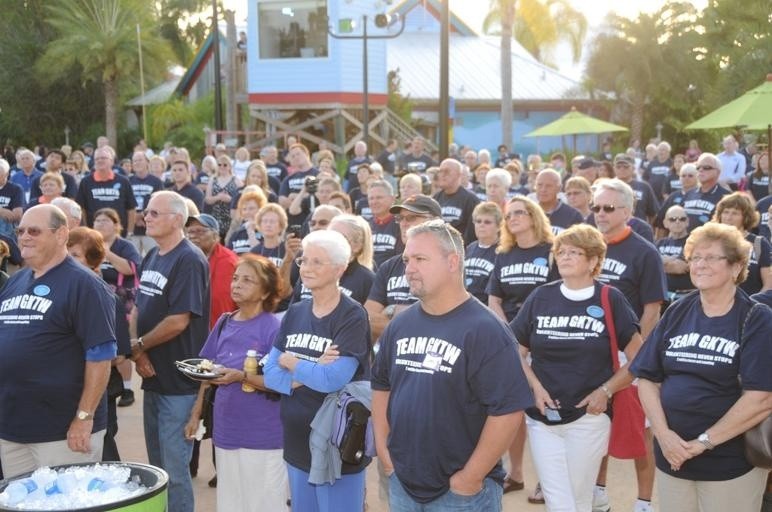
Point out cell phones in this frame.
[291,225,302,238]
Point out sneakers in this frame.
[593,497,610,512]
[118,390,134,406]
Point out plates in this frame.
[175,358,225,382]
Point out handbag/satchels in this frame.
[339,401,373,465]
[115,288,138,324]
[744,416,772,468]
[201,385,219,439]
[609,386,648,459]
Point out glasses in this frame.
[218,164,227,166]
[689,254,727,263]
[296,257,338,267]
[394,214,432,222]
[143,210,177,217]
[308,219,330,227]
[557,248,585,257]
[15,227,56,236]
[440,166,721,224]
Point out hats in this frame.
[185,213,219,231]
[390,194,441,218]
[573,153,633,168]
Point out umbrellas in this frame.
[523,107,630,159]
[684,72,772,194]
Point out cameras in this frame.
[305,177,319,193]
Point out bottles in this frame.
[0,472,122,505]
[241,349,258,392]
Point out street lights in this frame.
[322,10,406,152]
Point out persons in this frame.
[0,133,772,511]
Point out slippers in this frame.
[528,481,546,503]
[502,474,524,492]
